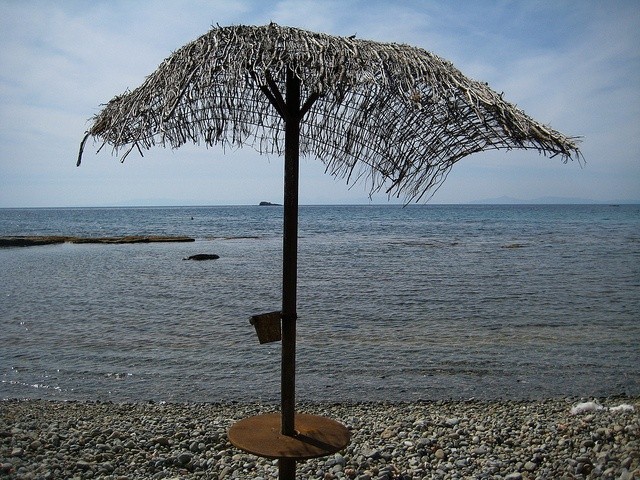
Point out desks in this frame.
[227,413,350,480]
